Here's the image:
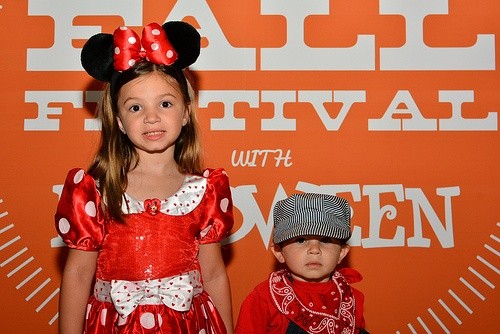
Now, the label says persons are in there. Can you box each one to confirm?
[54,21,234,334]
[234,193,372,334]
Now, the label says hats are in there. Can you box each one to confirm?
[273,193,351,244]
